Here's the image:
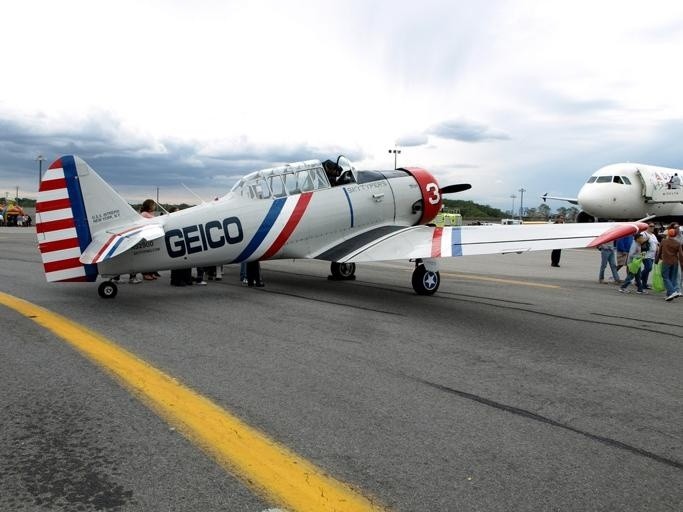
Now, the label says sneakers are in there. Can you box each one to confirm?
[112,271,160,283]
[599,279,653,295]
[665,291,682,301]
[197,272,224,285]
[243,278,264,288]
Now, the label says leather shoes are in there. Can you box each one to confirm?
[552,263,560,267]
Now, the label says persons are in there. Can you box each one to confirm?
[596,216,683,301]
[103,196,268,288]
[6,214,32,228]
[550,214,565,268]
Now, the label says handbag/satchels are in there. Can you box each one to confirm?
[642,240,650,252]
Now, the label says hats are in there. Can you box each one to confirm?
[668,229,675,236]
[633,234,643,240]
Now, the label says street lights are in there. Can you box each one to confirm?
[509,193,516,215]
[32,155,47,188]
[518,188,526,219]
[388,149,401,170]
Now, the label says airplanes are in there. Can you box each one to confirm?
[536,163,683,228]
[30,153,653,299]
